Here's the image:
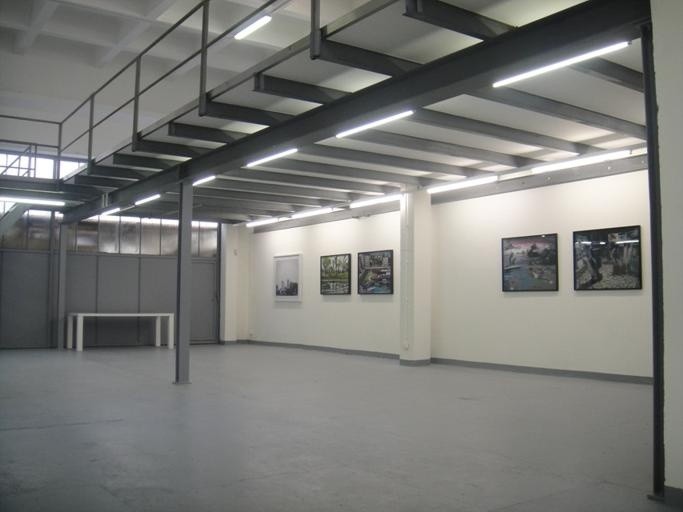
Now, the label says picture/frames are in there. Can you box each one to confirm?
[319,253,350,296]
[572,224,642,292]
[500,232,559,294]
[356,250,394,296]
[273,253,302,302]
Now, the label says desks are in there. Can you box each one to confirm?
[66,310,175,353]
